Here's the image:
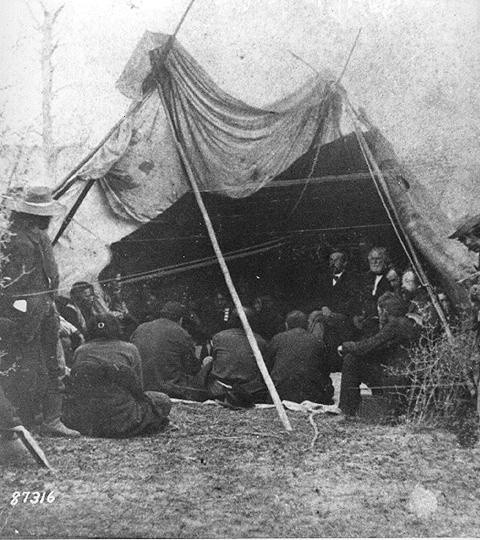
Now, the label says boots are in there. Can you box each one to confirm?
[39,391,81,439]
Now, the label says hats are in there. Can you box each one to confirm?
[6,182,67,217]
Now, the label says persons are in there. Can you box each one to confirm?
[56,264,269,439]
[267,246,452,423]
[2,186,81,439]
